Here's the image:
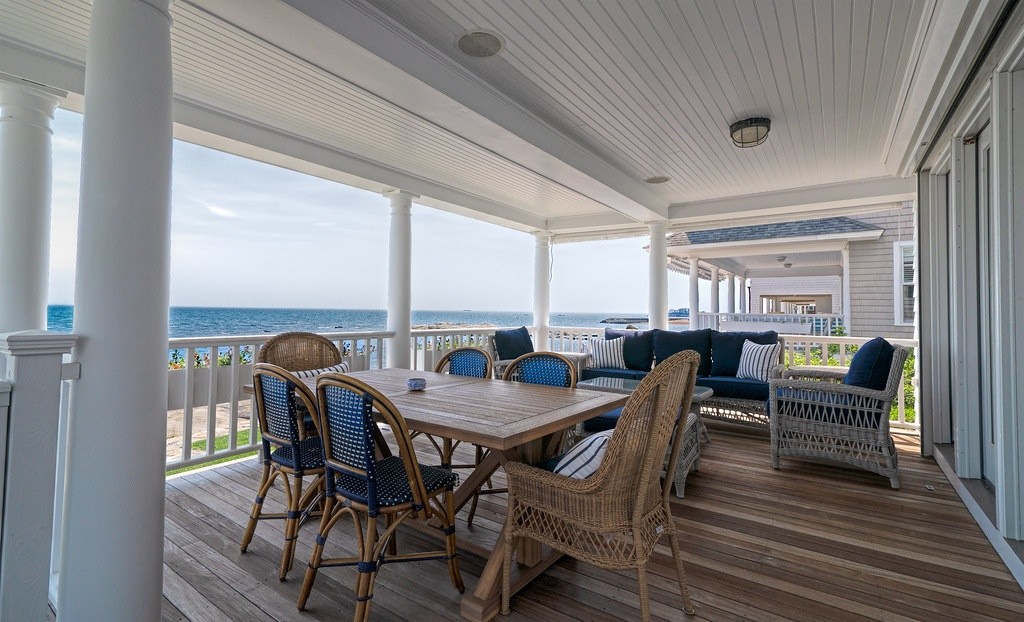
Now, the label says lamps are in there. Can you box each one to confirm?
[730,117,771,148]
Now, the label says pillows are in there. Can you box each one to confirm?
[556,427,616,480]
[736,338,782,383]
[589,335,628,371]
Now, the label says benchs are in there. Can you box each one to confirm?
[574,324,786,440]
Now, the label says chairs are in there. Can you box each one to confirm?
[498,349,702,622]
[486,327,586,451]
[769,336,908,491]
[240,331,398,583]
[399,345,492,506]
[466,351,577,527]
[298,372,466,622]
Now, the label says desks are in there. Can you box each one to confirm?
[242,368,632,622]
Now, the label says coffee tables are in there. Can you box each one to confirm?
[575,376,714,458]
